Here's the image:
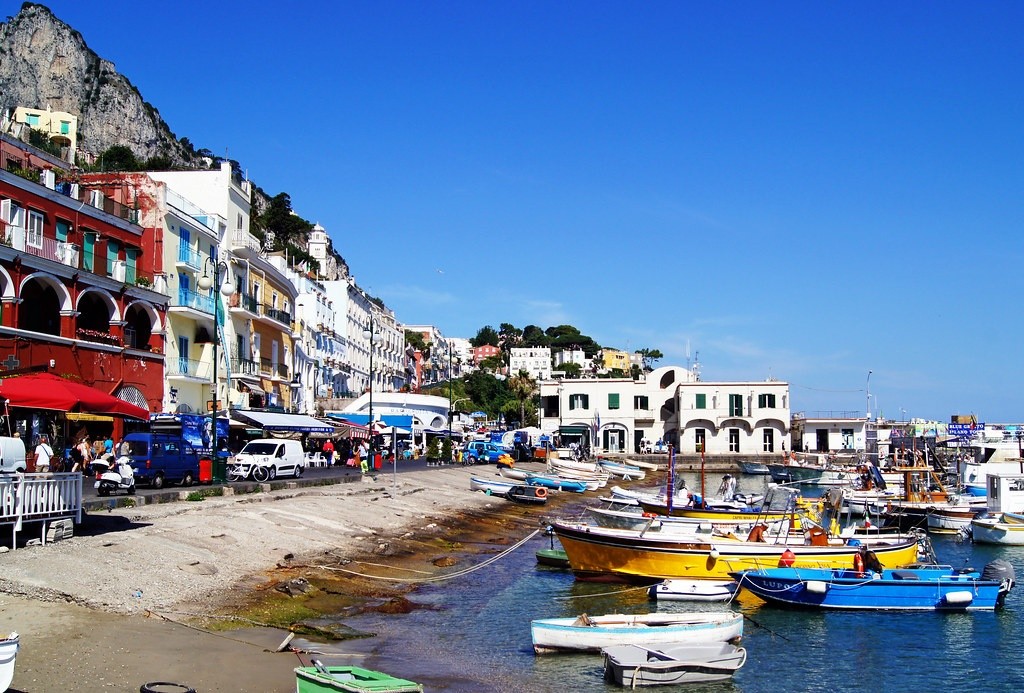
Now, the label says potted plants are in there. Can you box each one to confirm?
[135,275,150,289]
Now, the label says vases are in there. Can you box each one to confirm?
[76,336,118,345]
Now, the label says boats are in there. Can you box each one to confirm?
[534,434,1024,578]
[597,642,747,684]
[529,610,743,653]
[469,477,548,503]
[727,561,1001,614]
[290,647,425,692]
[647,578,740,601]
[498,454,660,493]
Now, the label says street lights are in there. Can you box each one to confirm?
[443,341,458,441]
[196,256,235,485]
[361,314,382,472]
[865,369,873,420]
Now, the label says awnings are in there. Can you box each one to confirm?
[238,411,334,433]
[242,380,265,396]
[561,429,582,436]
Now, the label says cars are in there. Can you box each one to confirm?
[462,441,509,465]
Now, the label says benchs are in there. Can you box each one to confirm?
[892,571,919,580]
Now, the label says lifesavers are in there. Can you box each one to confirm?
[642,513,657,517]
[853,553,864,578]
[535,488,547,497]
[515,487,525,495]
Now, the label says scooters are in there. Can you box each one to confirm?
[90,449,137,496]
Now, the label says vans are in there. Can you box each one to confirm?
[115,432,200,487]
[232,439,306,481]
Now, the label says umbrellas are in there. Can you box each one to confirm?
[0,373,149,421]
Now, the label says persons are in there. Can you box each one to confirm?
[640,436,663,455]
[554,438,558,447]
[782,440,785,450]
[71,435,122,472]
[686,493,711,510]
[805,442,809,453]
[33,437,54,479]
[353,439,369,468]
[477,446,490,464]
[574,444,590,462]
[409,444,420,460]
[323,440,334,468]
[451,442,469,466]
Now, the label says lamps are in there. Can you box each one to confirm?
[169,389,178,403]
[69,225,73,231]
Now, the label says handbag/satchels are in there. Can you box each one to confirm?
[83,467,93,476]
[70,445,83,463]
[116,442,122,457]
[111,445,116,458]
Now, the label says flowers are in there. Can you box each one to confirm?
[76,328,119,341]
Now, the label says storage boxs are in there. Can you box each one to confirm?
[92,459,110,471]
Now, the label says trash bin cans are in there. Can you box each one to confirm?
[372,451,382,470]
[216,456,228,479]
[200,456,213,485]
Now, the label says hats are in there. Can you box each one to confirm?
[41,438,46,443]
[687,493,692,498]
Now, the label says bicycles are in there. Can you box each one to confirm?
[224,451,270,483]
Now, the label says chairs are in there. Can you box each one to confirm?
[305,452,327,469]
